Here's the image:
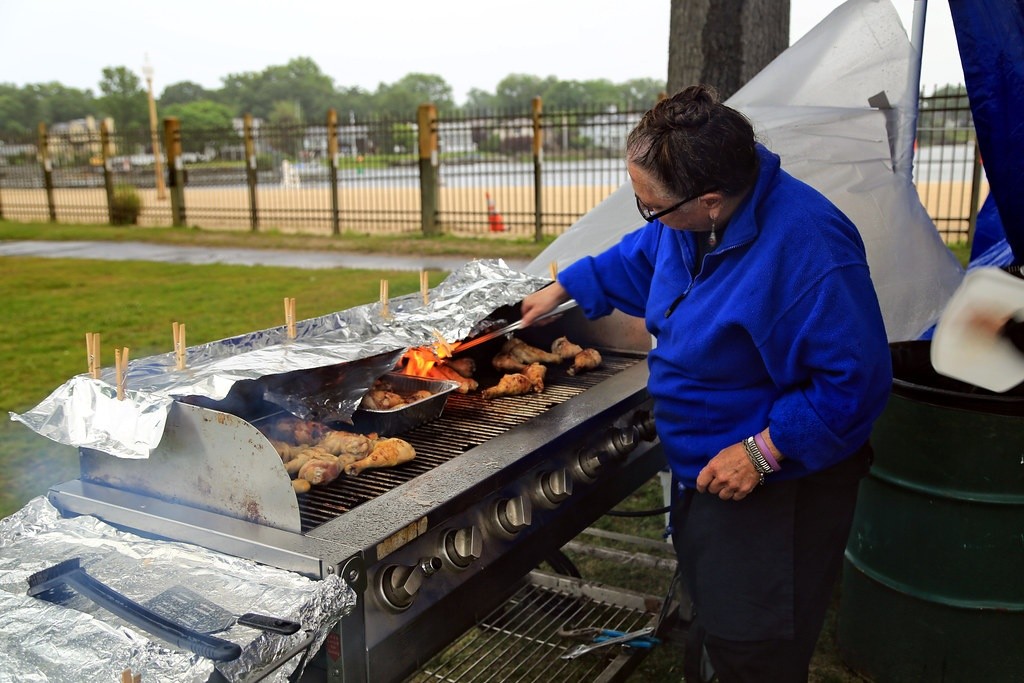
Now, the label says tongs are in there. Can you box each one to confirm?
[449,294,576,356]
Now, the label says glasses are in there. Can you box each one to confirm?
[635,186,726,224]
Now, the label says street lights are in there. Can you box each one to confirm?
[142,52,167,201]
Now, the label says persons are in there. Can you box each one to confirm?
[521,87,891,683]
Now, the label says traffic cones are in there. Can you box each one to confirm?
[485,191,510,235]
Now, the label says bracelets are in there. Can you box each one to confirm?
[742,433,781,485]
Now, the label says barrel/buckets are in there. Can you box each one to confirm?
[831,338,1024,683]
